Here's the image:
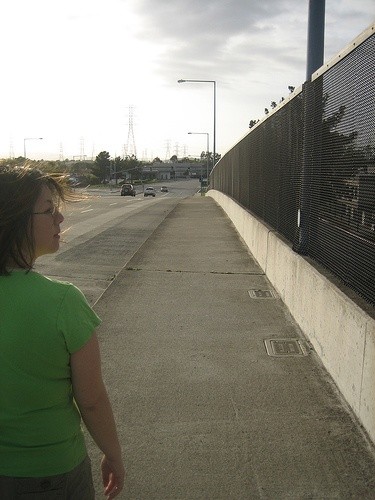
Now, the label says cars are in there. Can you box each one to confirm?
[144,187,156,197]
[160,186,168,192]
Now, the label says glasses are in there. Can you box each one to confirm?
[31,205,58,216]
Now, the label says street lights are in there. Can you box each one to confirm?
[178,79,216,165]
[187,133,209,185]
[23,138,44,158]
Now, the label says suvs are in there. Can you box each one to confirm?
[120,184,135,196]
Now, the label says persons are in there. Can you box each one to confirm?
[0,163,123,500]
